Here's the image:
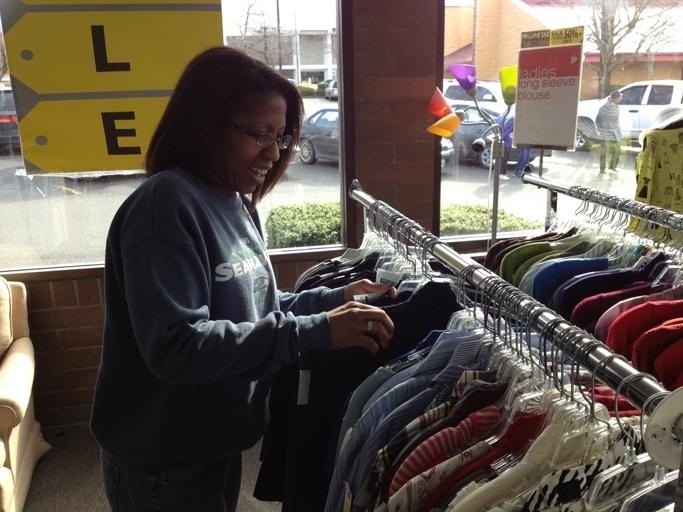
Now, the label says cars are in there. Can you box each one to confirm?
[297,73,530,173]
[0,81,23,158]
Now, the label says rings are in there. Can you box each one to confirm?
[366,319,376,334]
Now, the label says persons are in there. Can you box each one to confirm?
[89,46,399,510]
[593,90,625,178]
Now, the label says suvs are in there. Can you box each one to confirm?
[569,76,682,153]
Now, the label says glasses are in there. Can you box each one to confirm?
[228,120,293,151]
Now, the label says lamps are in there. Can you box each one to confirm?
[426,63,520,245]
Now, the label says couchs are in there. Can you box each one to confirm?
[1,274,52,512]
